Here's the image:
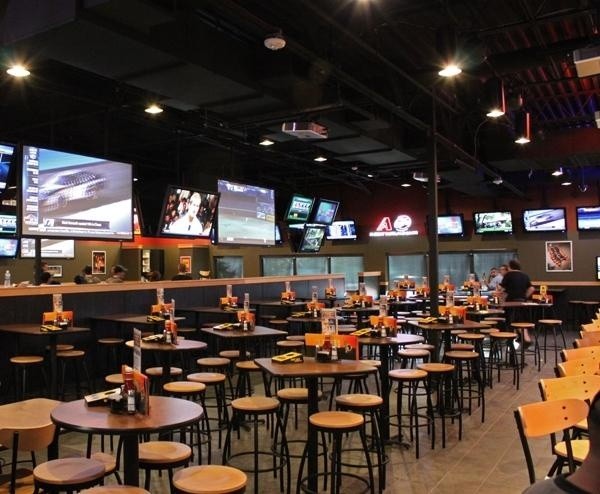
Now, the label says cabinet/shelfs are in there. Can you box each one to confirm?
[0,274,346,297]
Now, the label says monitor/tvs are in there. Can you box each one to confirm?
[214,174,277,249]
[19,238,36,261]
[520,208,567,234]
[0,235,18,259]
[438,213,465,237]
[310,197,340,226]
[1,214,16,234]
[324,220,359,242]
[471,210,514,236]
[16,140,135,243]
[156,184,221,240]
[295,225,325,252]
[40,238,75,260]
[282,193,315,224]
[576,205,600,231]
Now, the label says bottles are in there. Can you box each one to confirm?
[240,313,245,327]
[163,329,166,339]
[449,314,454,324]
[124,367,137,394]
[119,384,127,410]
[315,345,320,359]
[377,316,384,337]
[445,308,450,320]
[126,388,136,414]
[164,320,171,333]
[322,335,332,355]
[4,270,11,287]
[243,320,248,331]
[313,308,317,317]
[165,330,172,343]
[331,345,338,360]
[380,325,387,337]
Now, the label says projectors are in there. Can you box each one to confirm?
[413,172,442,186]
[279,121,329,141]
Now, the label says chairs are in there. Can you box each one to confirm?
[578,330,600,339]
[572,337,600,349]
[598,308,600,312]
[0,398,63,494]
[553,357,598,439]
[538,374,600,480]
[595,312,600,318]
[581,324,600,332]
[513,398,591,485]
[592,318,600,324]
[560,345,599,367]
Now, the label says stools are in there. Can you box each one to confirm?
[300,411,375,493]
[359,359,382,397]
[259,314,276,325]
[510,322,540,373]
[219,349,252,422]
[484,317,506,330]
[480,320,498,325]
[388,368,436,459]
[396,319,407,324]
[56,350,91,398]
[46,344,74,351]
[222,396,291,494]
[489,332,519,390]
[277,387,323,430]
[397,315,405,319]
[333,393,391,494]
[276,341,304,355]
[233,359,283,430]
[158,381,212,465]
[172,464,248,494]
[177,326,196,340]
[457,333,486,388]
[202,322,215,328]
[338,327,357,335]
[185,372,229,449]
[439,330,467,343]
[197,357,240,440]
[451,343,480,407]
[410,362,463,449]
[337,324,356,328]
[397,325,402,334]
[396,349,430,368]
[97,337,124,371]
[6,355,50,400]
[535,319,567,366]
[141,331,155,337]
[90,451,123,485]
[268,320,289,330]
[137,441,196,491]
[403,344,435,364]
[285,335,307,356]
[32,457,105,494]
[440,351,486,423]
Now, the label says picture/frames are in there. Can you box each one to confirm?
[179,256,191,273]
[545,241,573,273]
[92,251,106,274]
[596,256,600,280]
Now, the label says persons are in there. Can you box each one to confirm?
[500,258,535,343]
[170,193,203,233]
[40,260,48,271]
[496,264,508,291]
[104,264,127,283]
[520,389,600,494]
[171,263,192,280]
[483,267,503,291]
[38,272,51,286]
